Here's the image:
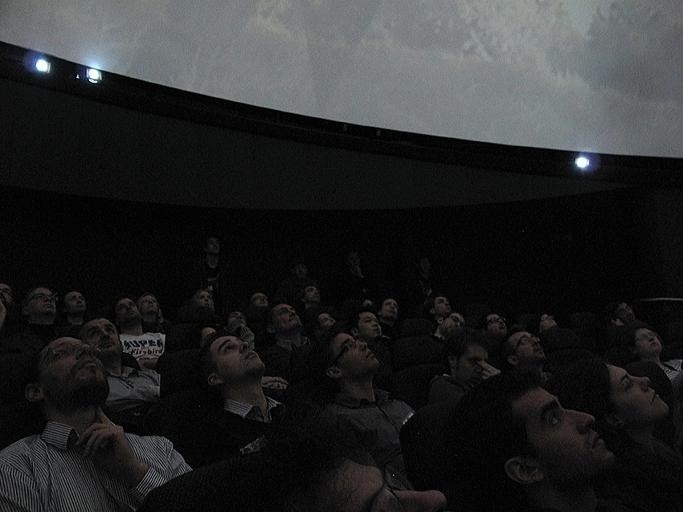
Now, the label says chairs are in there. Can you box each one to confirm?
[0,299,682,511]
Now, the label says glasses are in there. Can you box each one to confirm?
[333,338,365,364]
[367,466,409,512]
[487,317,506,324]
[513,335,540,352]
[24,293,55,307]
